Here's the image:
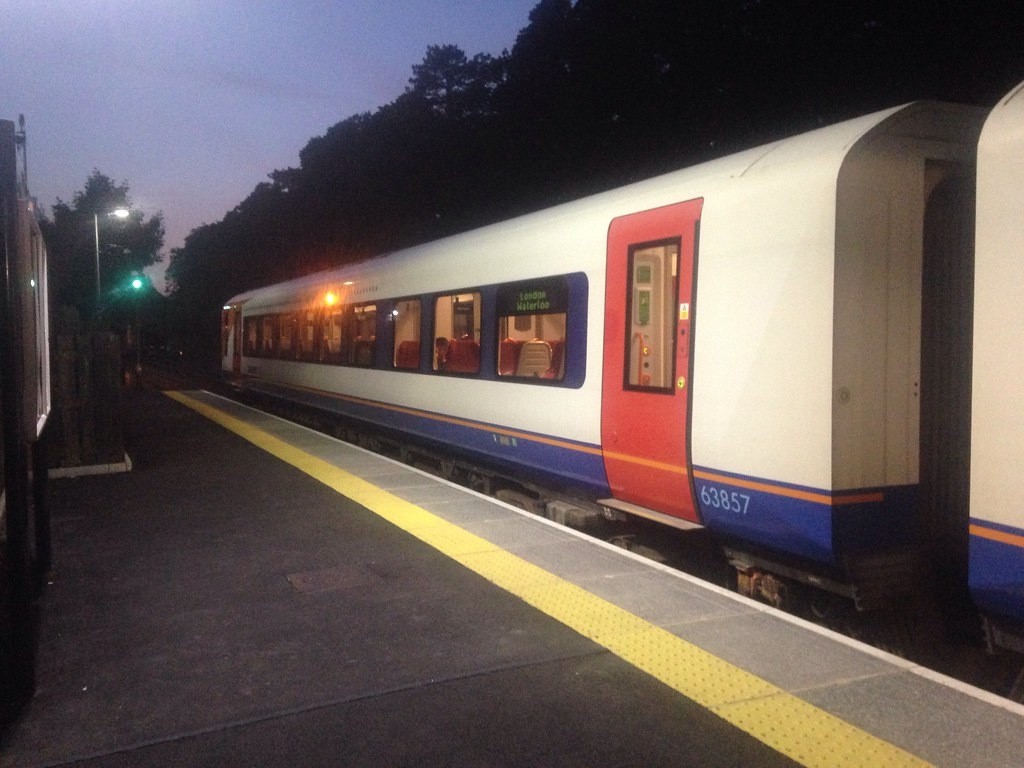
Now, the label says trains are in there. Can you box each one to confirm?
[222,78,1024,705]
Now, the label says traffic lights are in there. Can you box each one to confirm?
[133,277,145,314]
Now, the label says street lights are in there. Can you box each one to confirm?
[91,208,130,344]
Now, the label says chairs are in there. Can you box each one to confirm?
[501,339,524,375]
[513,341,553,378]
[444,341,478,373]
[247,340,373,365]
[397,341,420,369]
